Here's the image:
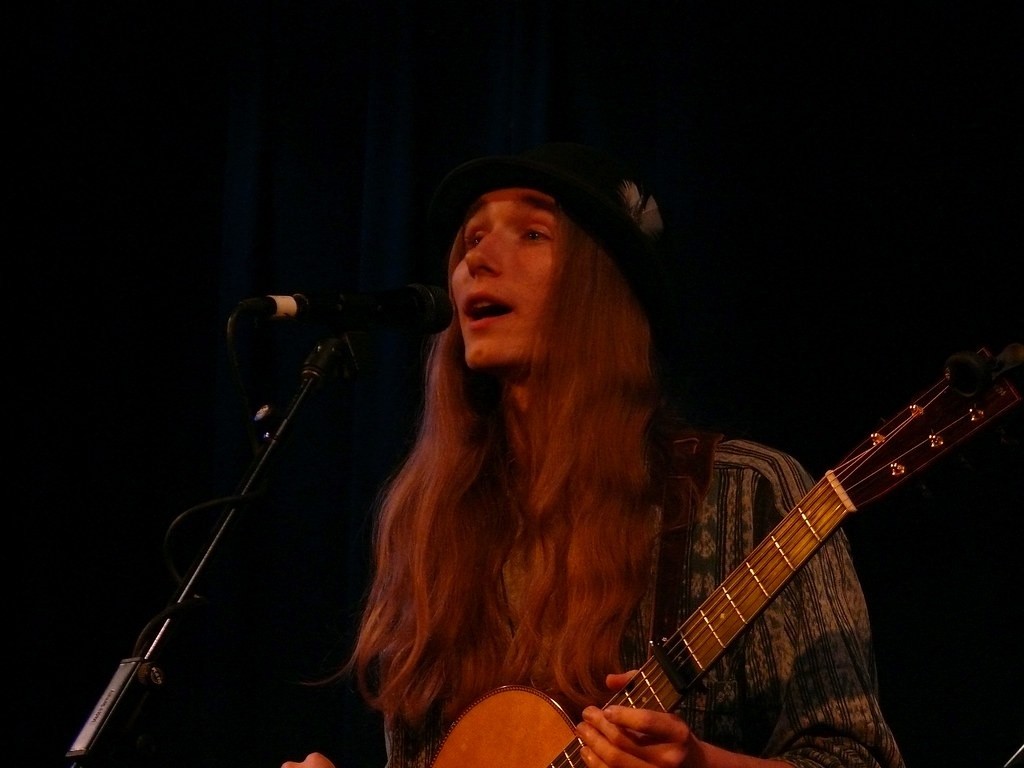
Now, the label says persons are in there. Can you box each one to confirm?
[276,142,906,768]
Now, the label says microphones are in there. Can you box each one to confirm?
[244,283,454,337]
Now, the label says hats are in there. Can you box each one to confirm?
[429,145,672,293]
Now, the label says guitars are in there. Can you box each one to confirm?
[427,332,1022,768]
